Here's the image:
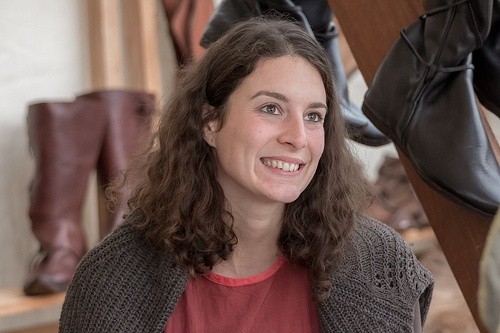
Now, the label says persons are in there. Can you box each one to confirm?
[58,16,437,333]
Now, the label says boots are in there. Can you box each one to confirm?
[199,0,393,147]
[23,101,108,295]
[361,0,500,218]
[76,90,156,242]
